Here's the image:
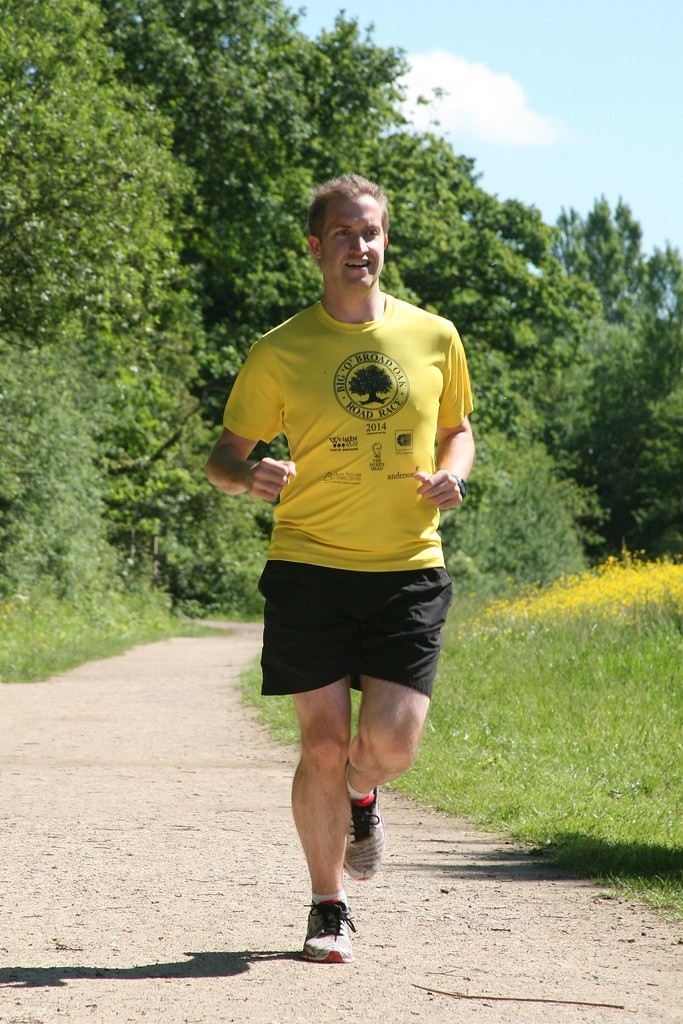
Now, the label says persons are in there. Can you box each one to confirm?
[203,171,479,964]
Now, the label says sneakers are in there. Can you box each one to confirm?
[302,901,355,964]
[342,756,385,881]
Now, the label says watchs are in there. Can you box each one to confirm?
[447,472,468,501]
